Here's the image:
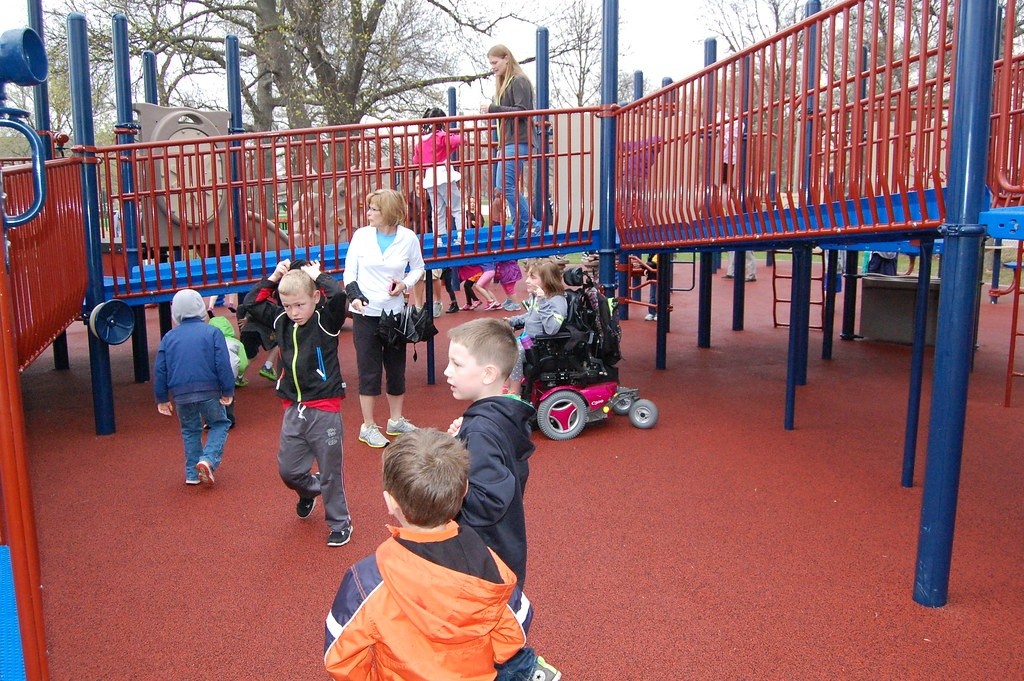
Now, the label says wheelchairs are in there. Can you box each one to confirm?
[509,267,660,442]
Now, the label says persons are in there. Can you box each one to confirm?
[699,105,745,216]
[444,318,538,592]
[153,289,235,485]
[824,250,916,292]
[644,254,657,321]
[322,427,562,681]
[244,258,353,546]
[504,258,568,398]
[721,250,757,282]
[482,44,542,239]
[202,292,280,433]
[412,107,468,247]
[405,172,619,318]
[618,135,662,227]
[343,188,426,448]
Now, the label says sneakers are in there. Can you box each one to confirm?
[386,416,419,435]
[327,526,353,545]
[296,471,320,518]
[359,423,390,447]
[234,373,248,387]
[259,365,277,380]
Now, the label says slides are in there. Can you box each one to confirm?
[240,205,353,319]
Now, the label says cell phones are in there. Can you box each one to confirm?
[387,281,396,291]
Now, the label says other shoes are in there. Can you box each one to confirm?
[207,306,215,318]
[186,479,200,484]
[470,298,482,310]
[745,277,757,282]
[501,298,521,310]
[644,314,657,320]
[484,300,501,310]
[505,225,541,237]
[196,458,214,484]
[526,655,562,681]
[228,302,237,312]
[459,303,470,310]
[446,302,459,313]
[433,301,443,317]
[722,274,734,280]
